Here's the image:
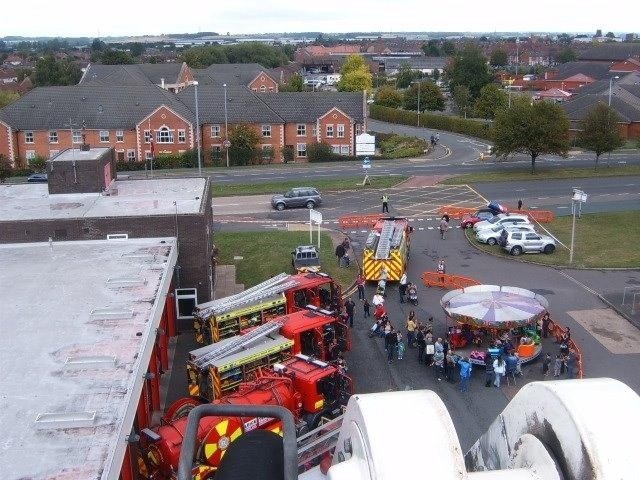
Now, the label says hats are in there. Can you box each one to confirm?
[302,71,376,106]
[461,207,499,229]
[28,172,47,182]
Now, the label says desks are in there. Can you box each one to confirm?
[507,77,516,109]
[569,189,588,261]
[223,83,231,167]
[608,76,621,121]
[193,81,203,171]
[411,81,420,127]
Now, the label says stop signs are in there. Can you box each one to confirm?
[362,214,412,285]
[186,303,354,404]
[192,265,351,346]
[136,350,352,479]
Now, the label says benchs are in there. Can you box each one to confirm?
[150,129,154,152]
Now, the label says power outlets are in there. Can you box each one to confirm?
[291,245,321,272]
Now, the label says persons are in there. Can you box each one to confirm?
[334,239,578,393]
[439,217,448,240]
[441,212,449,232]
[380,193,389,213]
[430,133,440,141]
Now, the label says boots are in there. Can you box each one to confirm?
[499,227,557,255]
[476,221,536,246]
[472,212,530,231]
[271,187,323,211]
